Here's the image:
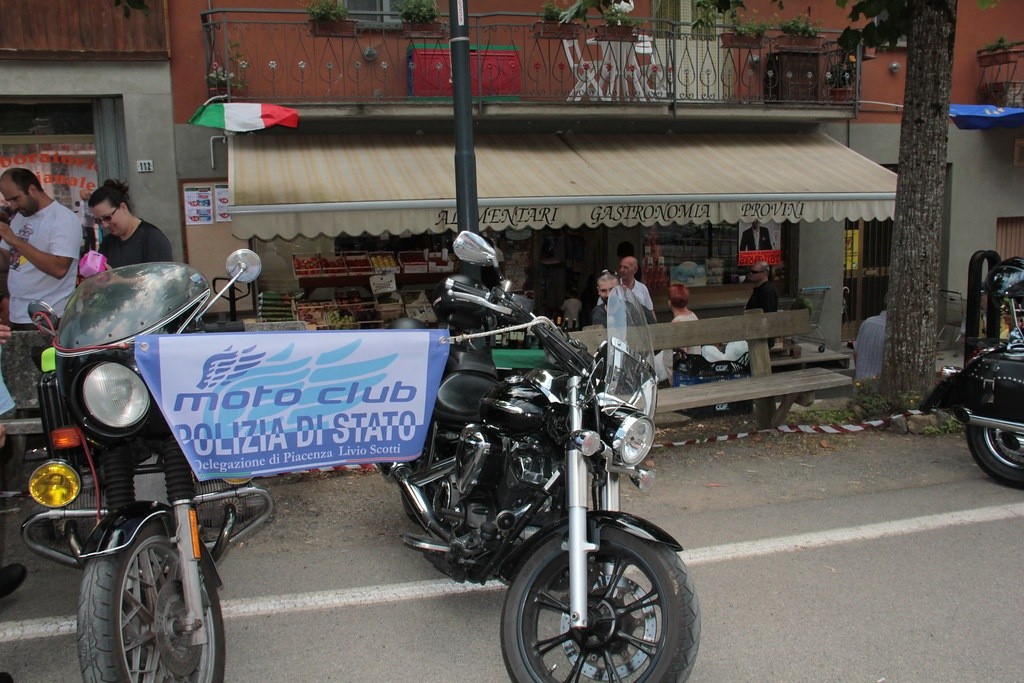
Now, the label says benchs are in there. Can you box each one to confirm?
[565,304,854,437]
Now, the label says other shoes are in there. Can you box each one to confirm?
[0,564,26,600]
[0,672,13,683]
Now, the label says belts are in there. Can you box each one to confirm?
[8,322,37,329]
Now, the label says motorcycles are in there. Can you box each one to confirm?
[918,249,1024,489]
[20,248,273,683]
[376,230,702,683]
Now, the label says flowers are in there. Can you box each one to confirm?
[202,59,231,84]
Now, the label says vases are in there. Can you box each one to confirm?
[206,85,232,104]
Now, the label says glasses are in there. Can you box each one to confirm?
[751,270,764,274]
[95,207,118,224]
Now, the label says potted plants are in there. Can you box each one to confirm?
[774,15,825,51]
[390,0,442,34]
[304,0,358,36]
[532,2,581,39]
[591,2,647,42]
[718,9,765,48]
[828,62,857,102]
[974,33,1023,67]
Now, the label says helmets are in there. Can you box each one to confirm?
[985,256,1024,315]
[432,274,489,324]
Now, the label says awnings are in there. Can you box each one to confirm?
[228,126,897,243]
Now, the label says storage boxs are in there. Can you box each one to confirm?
[289,251,455,329]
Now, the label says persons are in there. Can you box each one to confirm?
[740,220,772,251]
[0,324,28,683]
[523,267,538,293]
[89,179,181,336]
[561,290,582,330]
[571,284,592,321]
[0,168,83,330]
[619,256,657,324]
[81,192,102,259]
[662,284,701,387]
[745,261,778,349]
[590,273,642,329]
[853,291,888,394]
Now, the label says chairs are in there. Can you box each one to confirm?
[560,34,653,103]
